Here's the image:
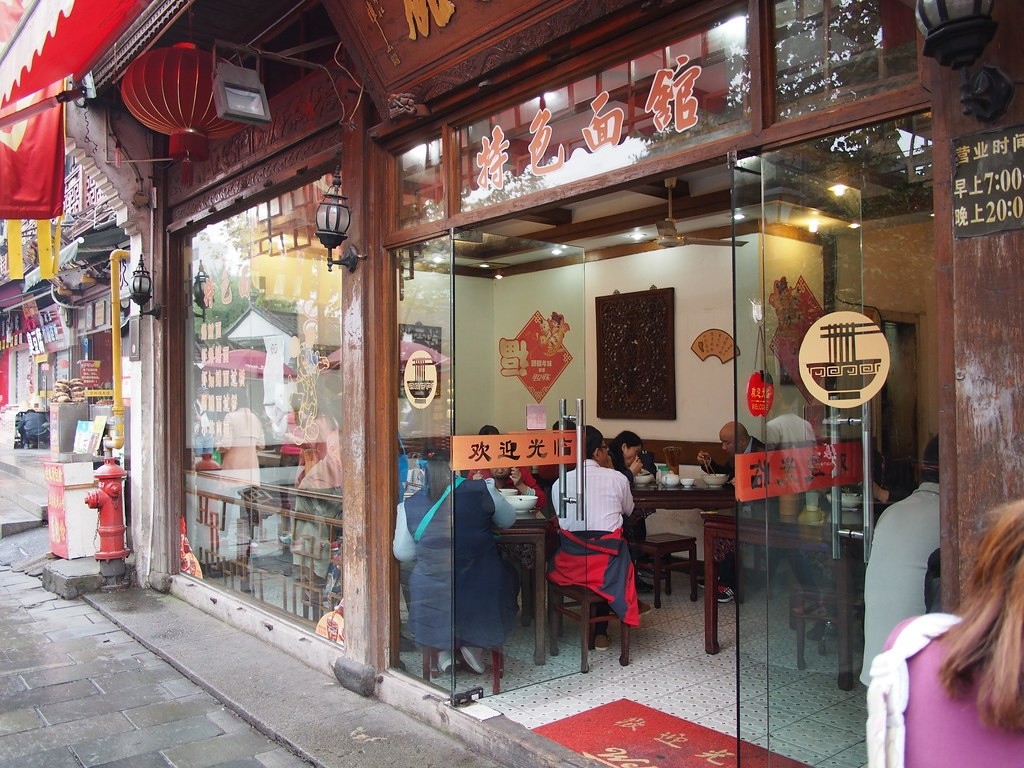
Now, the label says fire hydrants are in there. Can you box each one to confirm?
[84,456,132,592]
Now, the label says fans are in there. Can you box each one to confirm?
[605,176,748,252]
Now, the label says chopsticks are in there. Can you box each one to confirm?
[700,449,717,476]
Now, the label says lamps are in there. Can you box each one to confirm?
[126,253,161,321]
[211,39,353,127]
[915,0,1016,124]
[313,165,357,275]
[0,234,36,257]
[63,213,99,225]
[7,310,12,344]
[190,258,215,323]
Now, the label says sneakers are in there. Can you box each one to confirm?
[636,578,653,593]
[698,578,705,588]
[718,587,735,602]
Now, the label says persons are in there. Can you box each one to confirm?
[696,422,765,604]
[872,446,907,503]
[16,408,50,449]
[761,376,820,611]
[269,393,305,514]
[290,428,344,582]
[468,466,546,613]
[393,450,516,674]
[882,498,1024,768]
[285,388,342,546]
[607,430,654,594]
[552,425,652,650]
[193,387,214,457]
[214,387,266,544]
[477,424,500,436]
[858,433,942,689]
[537,420,576,479]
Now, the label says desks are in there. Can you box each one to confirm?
[236,478,295,593]
[700,500,886,691]
[622,485,735,547]
[491,510,547,666]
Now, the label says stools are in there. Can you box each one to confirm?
[793,577,868,672]
[422,636,504,695]
[632,533,697,609]
[547,582,630,674]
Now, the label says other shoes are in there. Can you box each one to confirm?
[436,649,460,674]
[23,443,29,448]
[461,645,485,674]
[594,635,609,651]
[806,619,838,656]
[640,600,651,617]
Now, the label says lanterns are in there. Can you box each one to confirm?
[120,41,248,159]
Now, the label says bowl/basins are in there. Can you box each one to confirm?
[701,474,729,487]
[497,489,538,513]
[825,492,863,508]
[680,479,695,488]
[633,475,654,486]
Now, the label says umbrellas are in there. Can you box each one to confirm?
[202,346,299,380]
[317,339,453,375]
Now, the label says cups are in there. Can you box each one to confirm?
[805,491,818,512]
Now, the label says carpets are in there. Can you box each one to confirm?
[528,697,818,768]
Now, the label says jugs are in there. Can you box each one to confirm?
[661,470,679,488]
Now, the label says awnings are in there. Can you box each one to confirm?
[0,0,183,220]
[22,228,131,295]
